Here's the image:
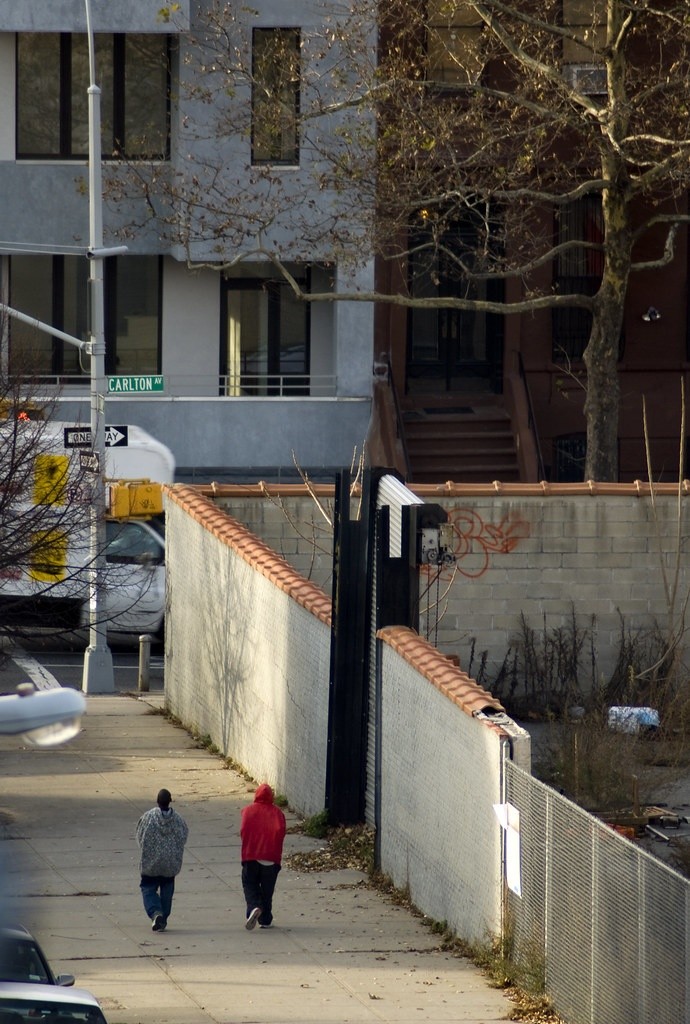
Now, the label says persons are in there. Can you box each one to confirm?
[136,789,188,932]
[240,784,287,930]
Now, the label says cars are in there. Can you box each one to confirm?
[1,922,76,987]
[1,982,107,1024]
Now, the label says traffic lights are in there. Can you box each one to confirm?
[108,486,130,518]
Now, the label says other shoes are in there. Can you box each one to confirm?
[246,907,262,930]
[260,922,272,928]
[157,927,165,931]
[152,914,164,931]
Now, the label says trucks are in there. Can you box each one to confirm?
[0,418,175,647]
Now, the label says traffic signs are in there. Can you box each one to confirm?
[63,426,129,448]
